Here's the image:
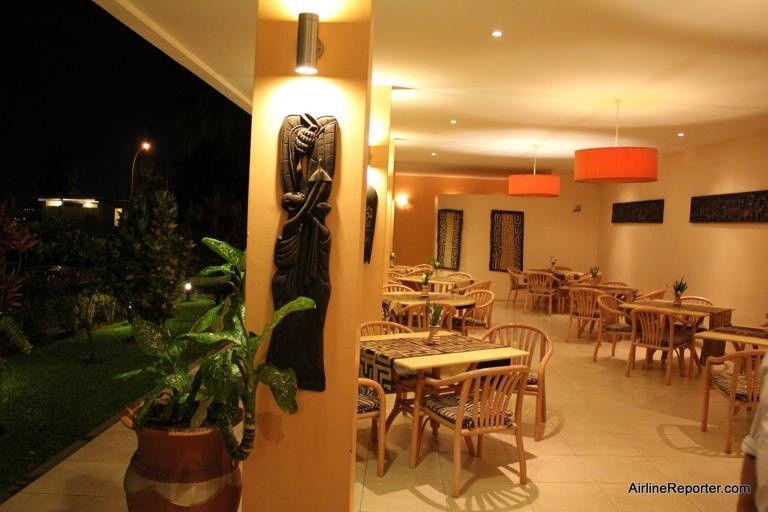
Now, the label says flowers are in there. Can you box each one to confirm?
[549,255,559,263]
[431,259,444,267]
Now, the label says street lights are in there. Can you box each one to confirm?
[129,140,154,197]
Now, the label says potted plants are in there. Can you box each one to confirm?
[109,236,318,512]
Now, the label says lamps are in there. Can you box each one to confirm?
[574,99,658,184]
[294,13,324,73]
[508,145,561,198]
